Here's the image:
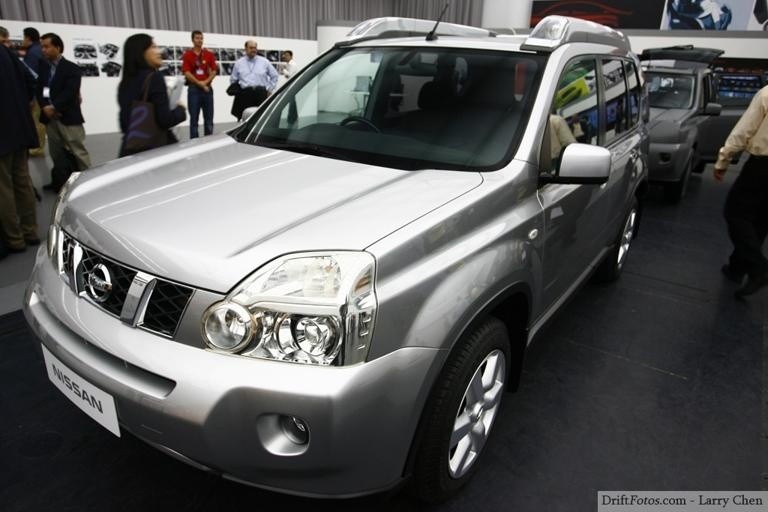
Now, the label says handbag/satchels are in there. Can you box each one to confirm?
[124,72,179,152]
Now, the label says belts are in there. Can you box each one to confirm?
[242,86,266,91]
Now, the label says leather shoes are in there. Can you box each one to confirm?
[720,264,744,284]
[733,258,768,299]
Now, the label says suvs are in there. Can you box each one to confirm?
[636,42,751,198]
[25,16,648,501]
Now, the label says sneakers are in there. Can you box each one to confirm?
[6,245,27,254]
[25,239,41,245]
[43,184,53,190]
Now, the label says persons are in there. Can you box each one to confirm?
[227,38,281,123]
[116,33,187,158]
[182,31,217,141]
[277,50,296,79]
[713,84,768,296]
[545,116,579,162]
[1,27,91,261]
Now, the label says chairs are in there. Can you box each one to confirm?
[386,80,451,146]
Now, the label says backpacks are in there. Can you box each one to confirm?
[51,164,73,194]
